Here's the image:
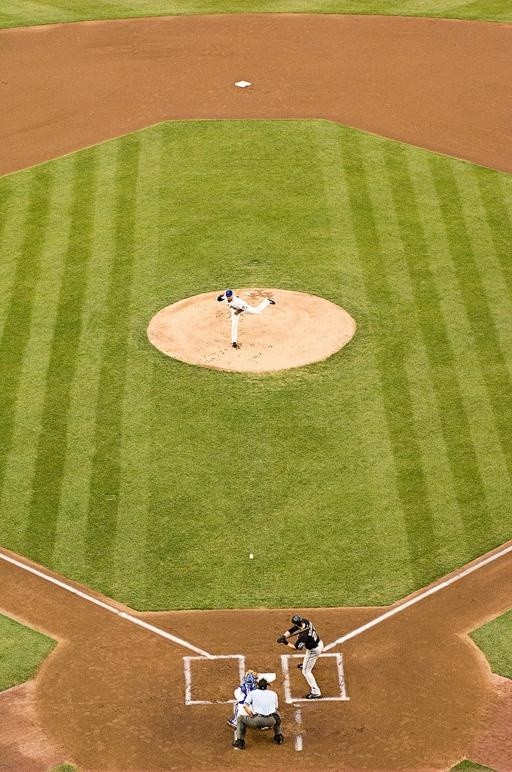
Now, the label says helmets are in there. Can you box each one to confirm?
[258,679,267,689]
[292,615,302,623]
[225,291,232,297]
[244,674,256,689]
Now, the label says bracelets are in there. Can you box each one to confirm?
[284,639,289,645]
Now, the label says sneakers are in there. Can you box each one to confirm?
[226,719,237,729]
[233,741,244,748]
[303,693,321,698]
[269,299,275,304]
[275,736,283,744]
[298,664,303,668]
[233,342,237,347]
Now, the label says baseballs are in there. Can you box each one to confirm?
[249,554,253,559]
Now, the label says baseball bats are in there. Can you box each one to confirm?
[284,627,310,639]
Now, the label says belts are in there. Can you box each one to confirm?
[257,713,271,717]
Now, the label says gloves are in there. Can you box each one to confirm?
[277,636,288,645]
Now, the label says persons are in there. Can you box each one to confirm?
[280,615,324,699]
[217,290,275,348]
[227,673,270,730]
[232,679,284,750]
